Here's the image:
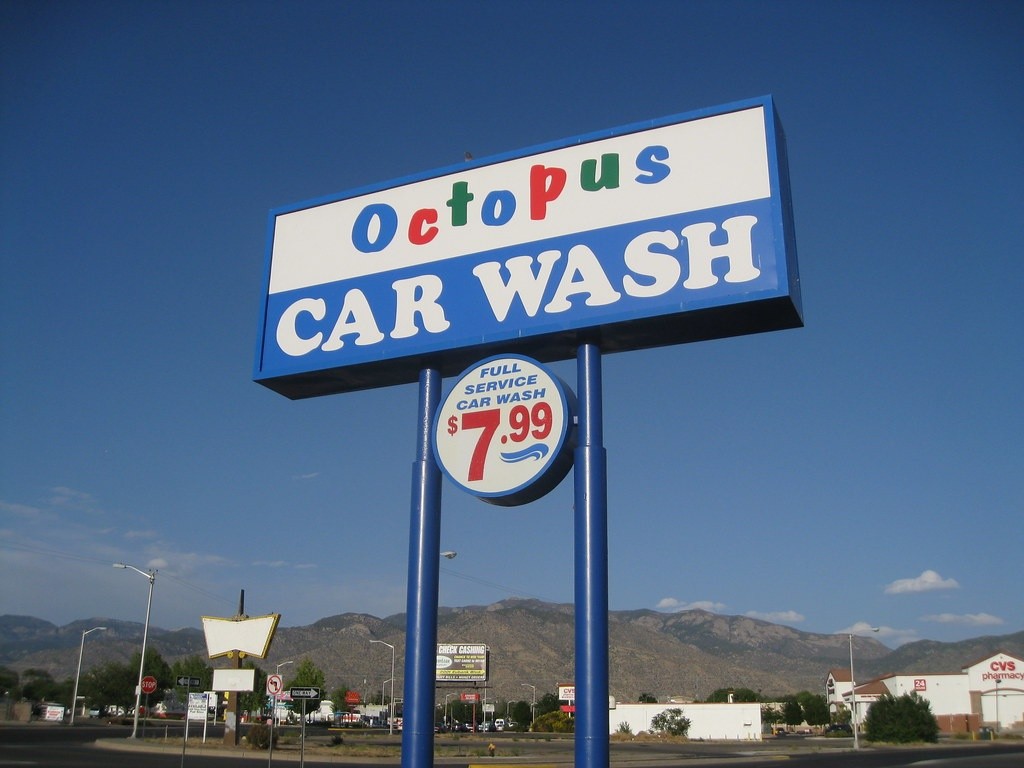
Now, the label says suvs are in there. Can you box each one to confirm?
[824,724,852,737]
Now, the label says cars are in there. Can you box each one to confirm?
[434,718,518,734]
[775,727,785,734]
[89,706,103,719]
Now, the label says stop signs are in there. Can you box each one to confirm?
[140,676,157,694]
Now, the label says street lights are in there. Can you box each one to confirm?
[275,661,294,728]
[69,626,107,727]
[112,563,158,739]
[849,627,880,750]
[994,678,1001,733]
[382,678,397,728]
[506,700,515,715]
[444,692,457,726]
[369,640,394,736]
[521,683,535,726]
[481,698,492,722]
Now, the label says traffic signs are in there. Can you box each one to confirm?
[177,675,201,687]
[290,687,320,699]
